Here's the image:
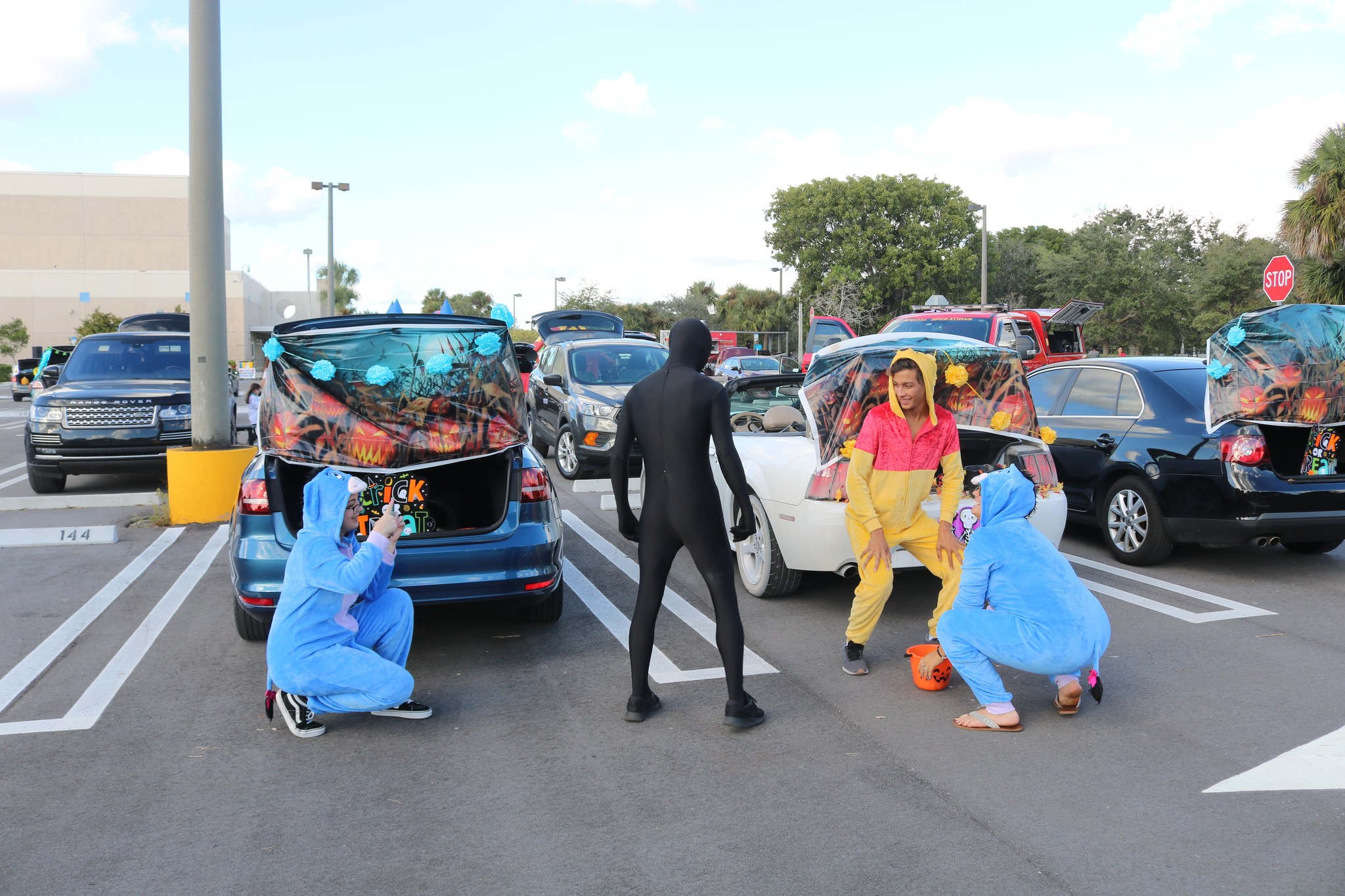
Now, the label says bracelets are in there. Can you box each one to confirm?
[937,646,947,659]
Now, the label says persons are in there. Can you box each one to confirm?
[1087,344,1101,358]
[746,340,762,356]
[703,364,722,376]
[266,467,433,737]
[610,318,765,726]
[841,348,966,675]
[245,358,270,446]
[1117,347,1127,357]
[918,462,1111,731]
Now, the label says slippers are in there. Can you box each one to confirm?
[953,708,1024,732]
[1054,693,1081,715]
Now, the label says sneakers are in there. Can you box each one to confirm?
[370,699,432,719]
[626,691,662,721]
[840,639,870,675]
[276,689,326,739]
[723,690,765,727]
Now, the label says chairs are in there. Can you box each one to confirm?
[758,405,803,433]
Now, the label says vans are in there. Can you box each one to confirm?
[803,295,1105,376]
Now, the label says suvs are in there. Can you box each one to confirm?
[24,311,238,495]
[525,309,669,480]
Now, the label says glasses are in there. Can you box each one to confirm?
[345,496,365,513]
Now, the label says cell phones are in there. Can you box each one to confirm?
[390,497,394,517]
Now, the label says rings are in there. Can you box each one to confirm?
[924,667,928,670]
[920,659,922,663]
[922,663,925,667]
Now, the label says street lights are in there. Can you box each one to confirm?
[513,293,522,330]
[554,277,566,311]
[312,181,349,317]
[303,248,312,318]
[771,268,782,298]
[525,321,532,331]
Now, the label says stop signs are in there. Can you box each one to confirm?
[1263,255,1294,302]
[809,306,815,326]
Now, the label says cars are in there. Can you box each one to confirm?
[702,346,807,404]
[512,342,544,400]
[997,304,1345,568]
[29,346,76,407]
[11,358,42,402]
[709,329,1068,601]
[227,313,564,642]
[227,365,239,397]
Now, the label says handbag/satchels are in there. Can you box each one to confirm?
[1300,421,1342,476]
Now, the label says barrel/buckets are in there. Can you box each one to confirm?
[904,644,952,690]
[964,464,1004,498]
